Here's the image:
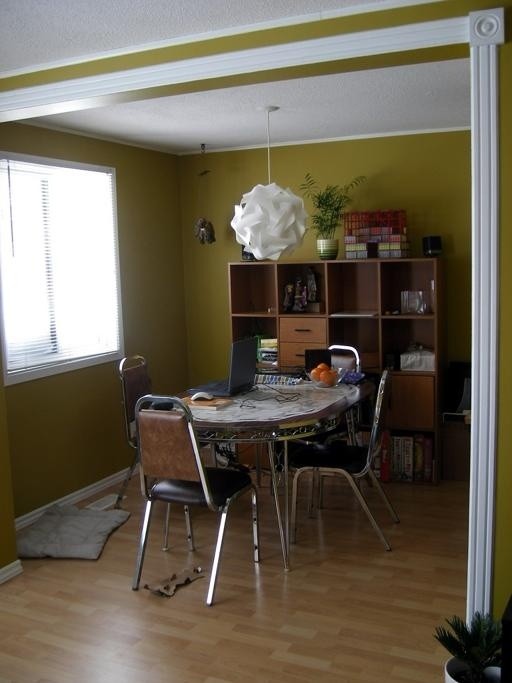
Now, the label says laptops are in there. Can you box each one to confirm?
[187,336,259,397]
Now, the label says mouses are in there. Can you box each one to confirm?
[190,391,214,401]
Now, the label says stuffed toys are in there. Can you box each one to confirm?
[193,216,217,243]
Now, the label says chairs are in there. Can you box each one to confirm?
[129,394,261,607]
[269,344,360,495]
[290,367,398,551]
[115,354,149,510]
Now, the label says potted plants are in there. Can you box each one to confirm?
[432,612,503,683]
[298,168,370,260]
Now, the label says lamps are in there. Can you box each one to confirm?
[231,105,308,263]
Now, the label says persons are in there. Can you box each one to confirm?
[305,265,318,302]
[292,276,304,311]
[282,280,295,310]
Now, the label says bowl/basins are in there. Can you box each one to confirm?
[341,370,366,385]
[305,367,347,387]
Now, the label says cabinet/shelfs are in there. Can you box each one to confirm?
[227,258,443,486]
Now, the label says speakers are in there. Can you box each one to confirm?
[305,349,331,371]
[422,235,443,256]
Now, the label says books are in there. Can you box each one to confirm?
[174,395,233,410]
[259,336,278,365]
[347,428,434,483]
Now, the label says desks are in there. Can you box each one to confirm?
[165,376,362,572]
